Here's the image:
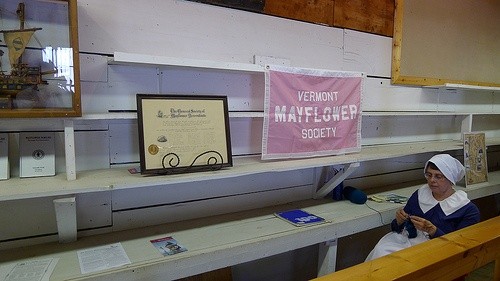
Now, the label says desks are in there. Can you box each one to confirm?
[0,171,500,281]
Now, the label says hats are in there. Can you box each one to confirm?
[424,154,465,185]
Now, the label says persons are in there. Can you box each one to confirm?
[363,152,482,281]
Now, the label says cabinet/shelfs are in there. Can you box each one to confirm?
[0,52,500,241]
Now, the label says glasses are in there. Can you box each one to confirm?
[425,173,446,182]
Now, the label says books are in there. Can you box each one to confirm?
[273,209,326,227]
